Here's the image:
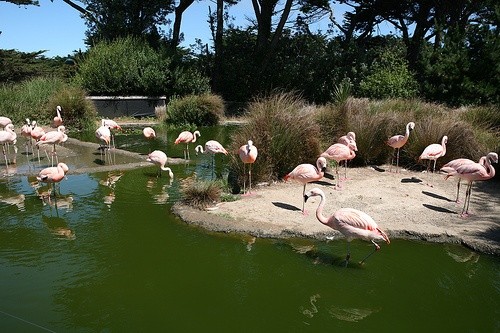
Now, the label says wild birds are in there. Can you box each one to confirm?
[418,134,448,188]
[93,126,111,157]
[319,141,357,190]
[101,118,123,151]
[20,117,34,158]
[0,123,18,159]
[440,155,491,205]
[443,151,499,219]
[35,162,70,196]
[194,140,228,180]
[337,131,356,181]
[234,139,258,197]
[30,121,50,162]
[142,150,174,179]
[303,187,390,270]
[39,132,68,162]
[142,127,157,140]
[0,115,13,131]
[173,129,201,160]
[283,156,328,216]
[384,121,416,174]
[53,105,62,127]
[34,125,66,167]
